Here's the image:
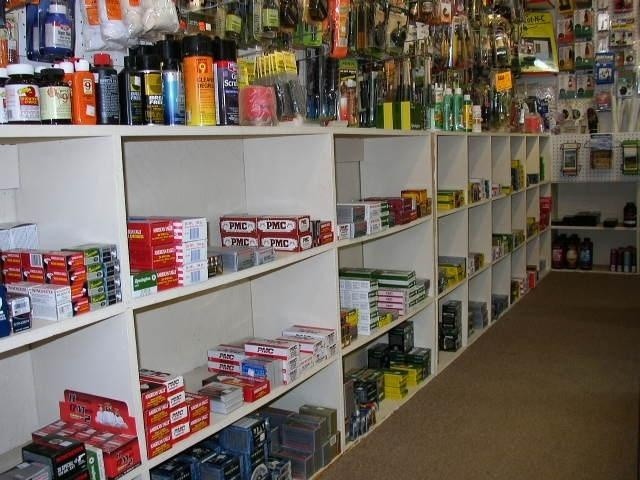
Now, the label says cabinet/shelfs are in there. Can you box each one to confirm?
[432,131,491,377]
[2,125,343,479]
[552,223,640,275]
[491,133,552,325]
[334,128,435,429]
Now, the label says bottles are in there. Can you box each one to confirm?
[0,1,238,127]
[552,233,638,274]
[385,57,490,133]
[624,200,637,227]
